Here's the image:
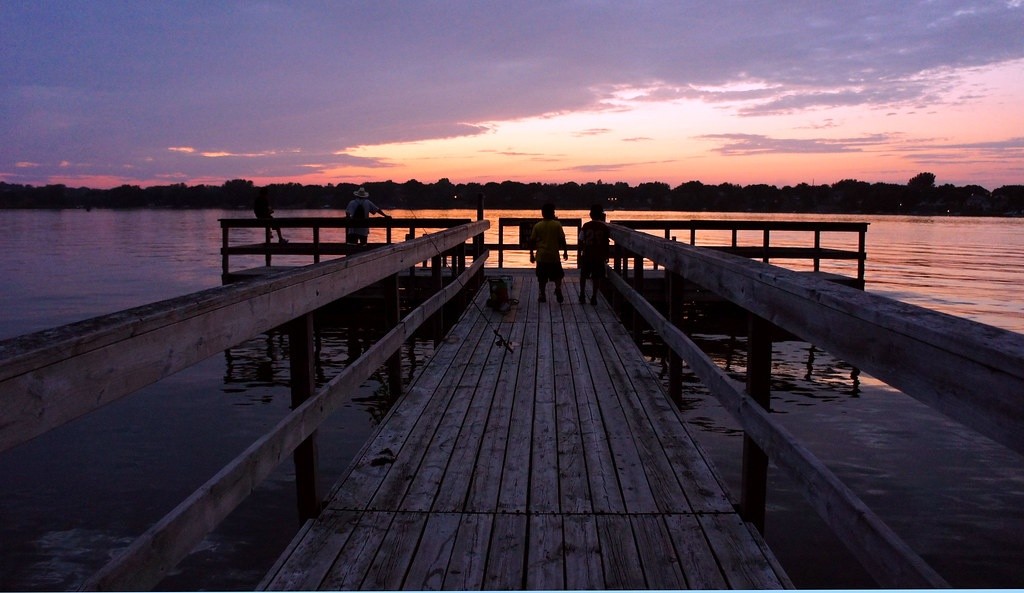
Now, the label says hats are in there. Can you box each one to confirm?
[354,189,369,198]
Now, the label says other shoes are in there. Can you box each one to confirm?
[538,296,546,302]
[555,288,564,302]
[579,295,587,304]
[590,298,597,305]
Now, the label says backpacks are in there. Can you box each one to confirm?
[353,199,366,218]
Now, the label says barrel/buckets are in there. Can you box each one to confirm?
[488,275,513,305]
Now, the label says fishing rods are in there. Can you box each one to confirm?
[406,203,514,355]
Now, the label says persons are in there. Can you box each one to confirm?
[346,187,392,256]
[528,203,568,303]
[253,188,289,244]
[577,206,609,305]
